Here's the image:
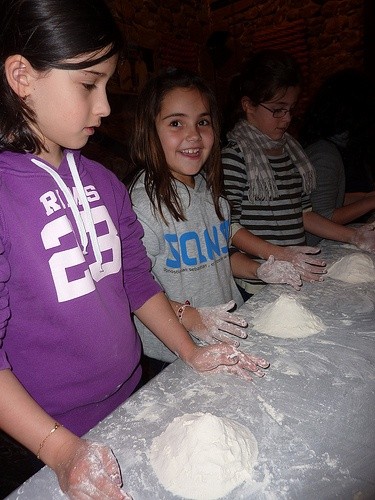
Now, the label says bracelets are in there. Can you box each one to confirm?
[36,422,64,461]
[176,300,190,323]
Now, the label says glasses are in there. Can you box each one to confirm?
[250,98,298,118]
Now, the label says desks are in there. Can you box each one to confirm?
[4,223,375,500]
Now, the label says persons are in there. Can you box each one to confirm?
[125,72,302,382]
[0,0,270,500]
[81,129,130,180]
[296,69,375,246]
[221,49,375,283]
